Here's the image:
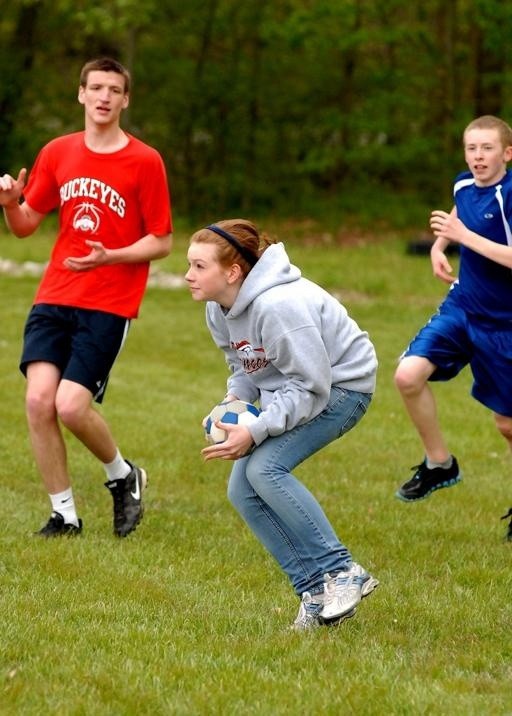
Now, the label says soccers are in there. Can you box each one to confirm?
[205,400,260,458]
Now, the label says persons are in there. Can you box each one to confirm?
[390,111,510,547]
[2,56,172,544]
[180,215,381,632]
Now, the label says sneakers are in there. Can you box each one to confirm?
[284,563,380,632]
[28,511,84,539]
[394,454,464,504]
[101,458,150,539]
